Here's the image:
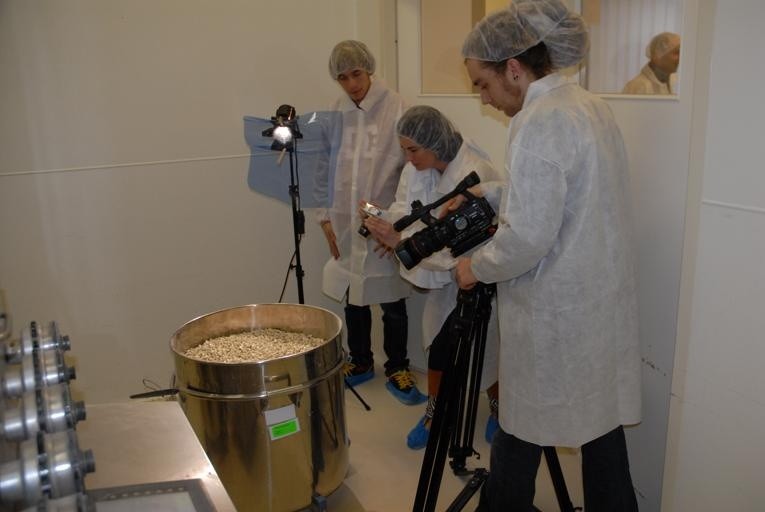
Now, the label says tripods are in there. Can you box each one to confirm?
[411,288,578,512]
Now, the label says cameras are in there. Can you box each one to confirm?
[358,203,382,238]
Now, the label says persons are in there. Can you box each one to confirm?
[358,105,502,449]
[622,32,680,95]
[313,40,420,405]
[440,10,641,512]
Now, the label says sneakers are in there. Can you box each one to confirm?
[385,369,421,405]
[344,358,374,389]
[407,406,434,449]
[485,400,499,443]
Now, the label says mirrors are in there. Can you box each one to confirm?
[414,0,519,99]
[573,0,686,101]
